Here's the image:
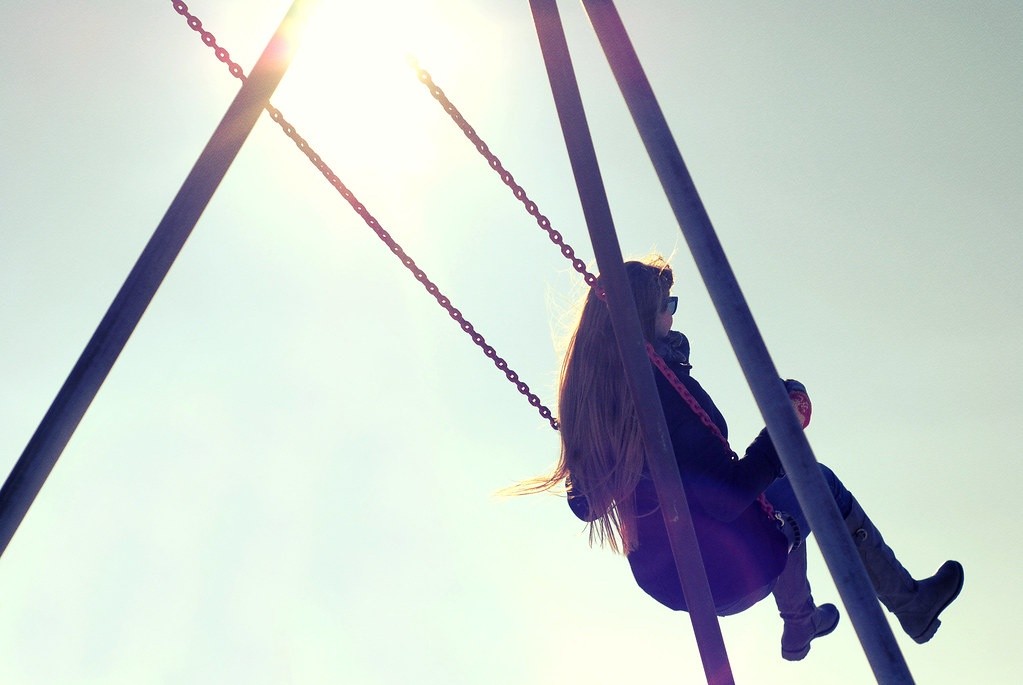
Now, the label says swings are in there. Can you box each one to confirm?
[169,0,803,617]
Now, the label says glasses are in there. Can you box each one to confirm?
[655,297,678,314]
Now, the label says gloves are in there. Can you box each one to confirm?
[780,378,811,430]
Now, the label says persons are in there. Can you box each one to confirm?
[489,261,965,661]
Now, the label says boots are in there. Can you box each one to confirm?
[773,535,840,661]
[845,496,964,645]
